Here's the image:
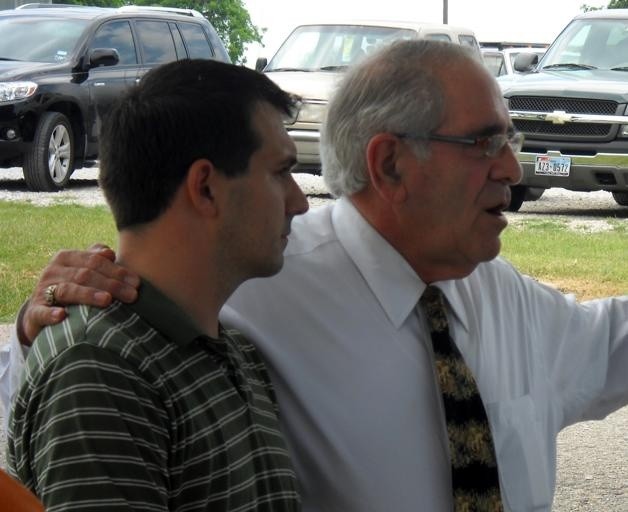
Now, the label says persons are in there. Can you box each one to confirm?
[15,39,628,507]
[6,57,309,511]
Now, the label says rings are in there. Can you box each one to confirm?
[41,279,60,307]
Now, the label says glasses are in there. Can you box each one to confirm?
[396,131,525,159]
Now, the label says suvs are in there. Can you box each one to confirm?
[0,0,236,193]
[495,8,628,209]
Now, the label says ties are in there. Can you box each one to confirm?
[420,285,504,512]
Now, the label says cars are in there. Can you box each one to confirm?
[254,21,484,172]
[480,44,580,77]
